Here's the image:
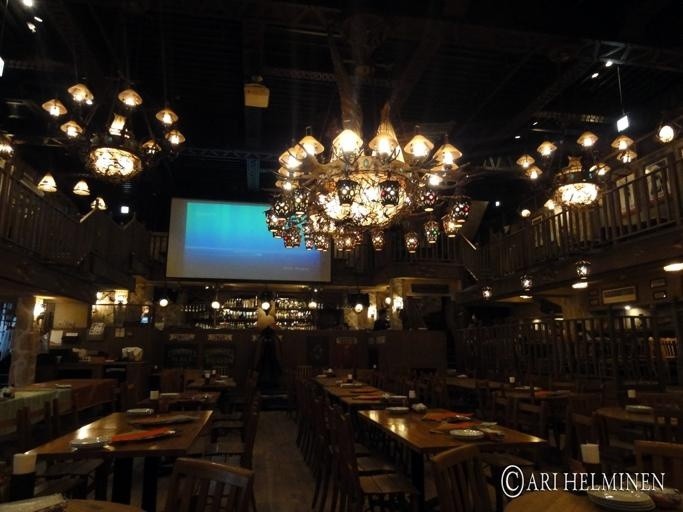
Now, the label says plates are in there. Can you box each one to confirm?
[517,386,542,391]
[69,439,111,449]
[625,405,654,414]
[316,367,426,416]
[585,486,657,512]
[126,408,153,415]
[160,392,180,398]
[56,385,71,388]
[447,428,484,442]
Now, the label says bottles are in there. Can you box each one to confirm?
[7,472,37,500]
[204,373,216,384]
[627,398,637,405]
[170,335,234,364]
[2,384,16,398]
[579,460,605,489]
[182,296,260,329]
[276,298,316,330]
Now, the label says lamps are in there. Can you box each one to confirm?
[36,79,186,214]
[0,133,16,160]
[516,112,675,212]
[575,259,591,283]
[481,282,494,301]
[267,61,489,255]
[520,272,536,293]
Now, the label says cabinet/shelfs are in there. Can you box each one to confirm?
[152,286,319,328]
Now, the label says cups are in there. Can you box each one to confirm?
[158,398,168,412]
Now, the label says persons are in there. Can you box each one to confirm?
[650,165,663,195]
[589,319,612,362]
[373,309,390,331]
[400,309,415,332]
